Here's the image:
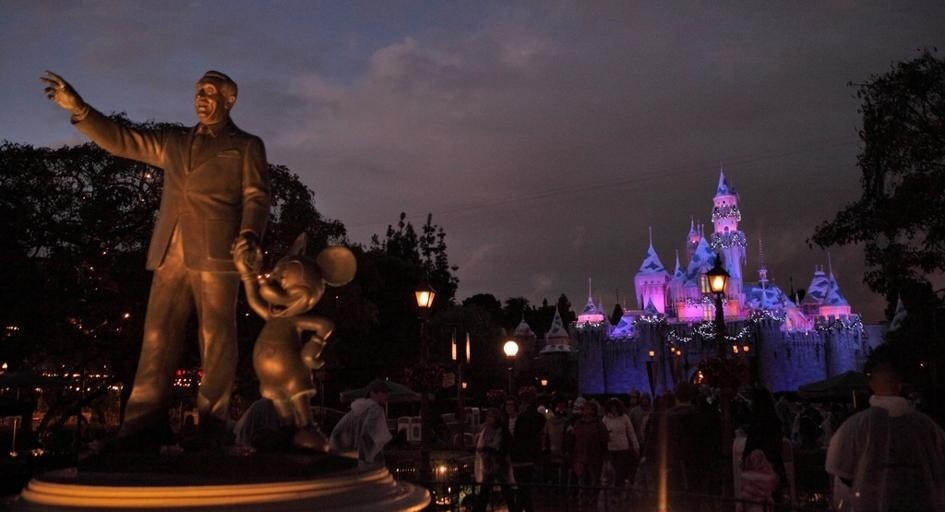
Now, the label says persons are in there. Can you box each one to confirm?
[731,367,940,512]
[473,373,676,512]
[333,380,393,470]
[40,70,271,461]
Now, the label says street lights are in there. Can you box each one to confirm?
[649,346,683,512]
[414,270,437,489]
[503,340,519,397]
[705,252,734,497]
[733,344,750,362]
[541,377,548,393]
